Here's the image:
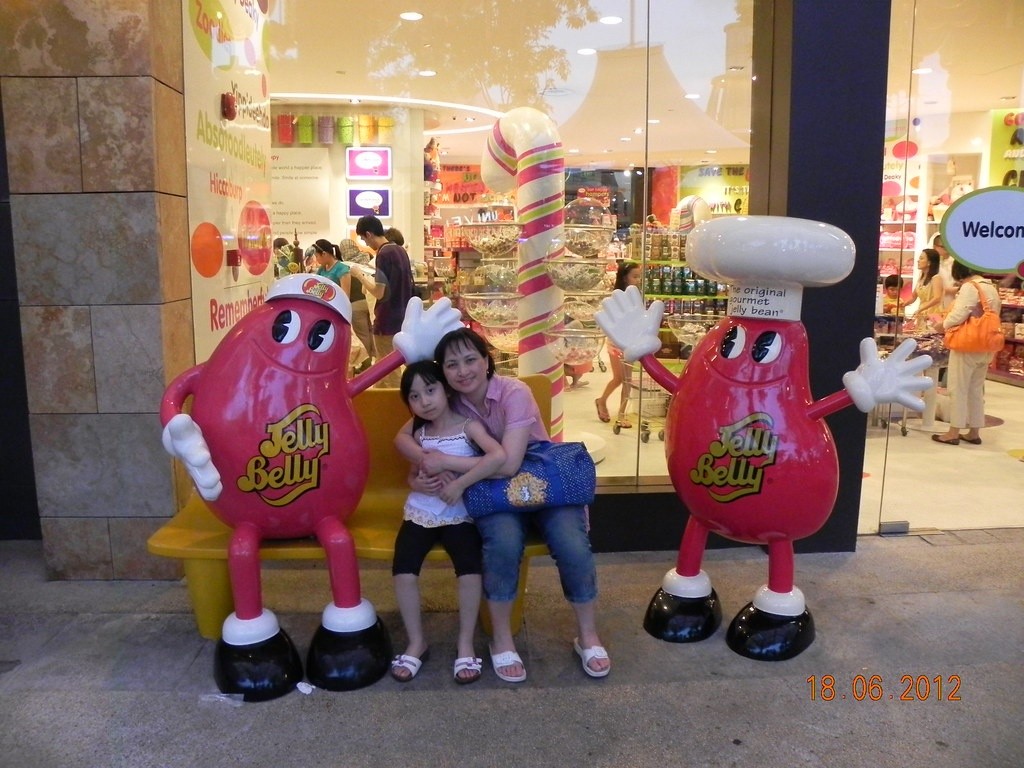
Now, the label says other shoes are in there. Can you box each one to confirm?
[959,434,981,444]
[354,364,372,375]
[932,434,959,445]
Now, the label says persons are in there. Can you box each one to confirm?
[883,235,961,382]
[274,214,416,374]
[595,262,641,428]
[391,328,611,683]
[934,259,1001,445]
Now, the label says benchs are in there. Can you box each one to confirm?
[148,374,555,640]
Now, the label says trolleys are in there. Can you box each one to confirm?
[620,355,666,443]
[872,402,912,437]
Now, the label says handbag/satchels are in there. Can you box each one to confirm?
[944,280,1004,351]
[413,285,429,300]
[462,440,596,519]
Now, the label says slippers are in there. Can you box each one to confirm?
[489,640,526,682]
[574,637,610,677]
[390,648,430,682]
[453,651,482,685]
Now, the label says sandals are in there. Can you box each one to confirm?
[621,421,632,428]
[595,398,610,422]
